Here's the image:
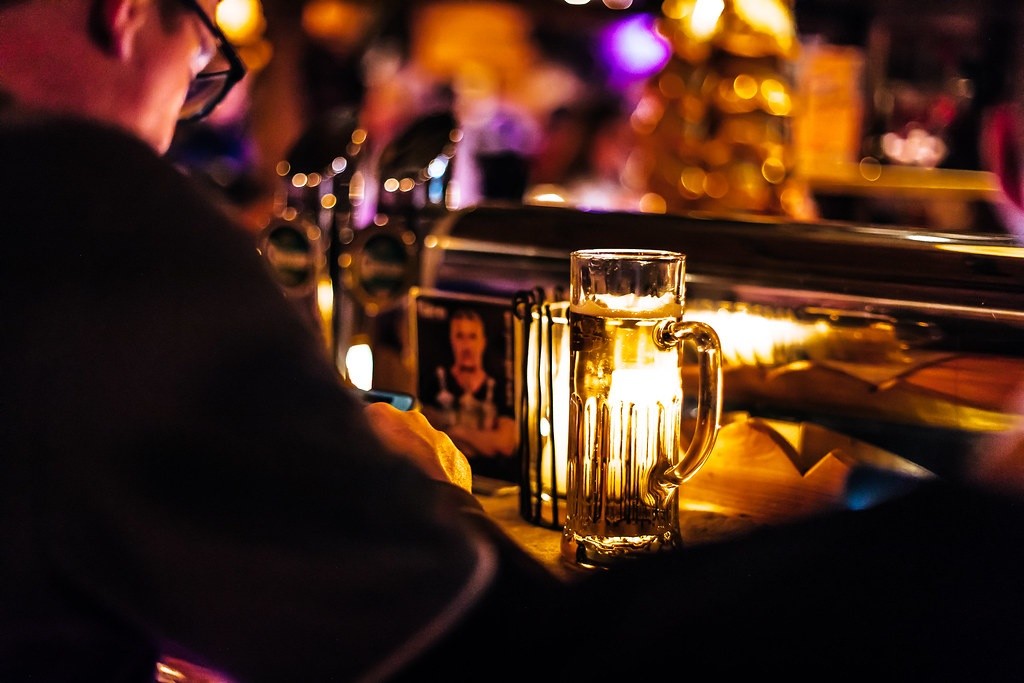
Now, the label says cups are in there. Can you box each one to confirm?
[559,249,725,573]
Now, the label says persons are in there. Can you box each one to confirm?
[417,308,517,461]
[1,1,608,683]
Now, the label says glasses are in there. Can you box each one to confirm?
[176,0,248,126]
